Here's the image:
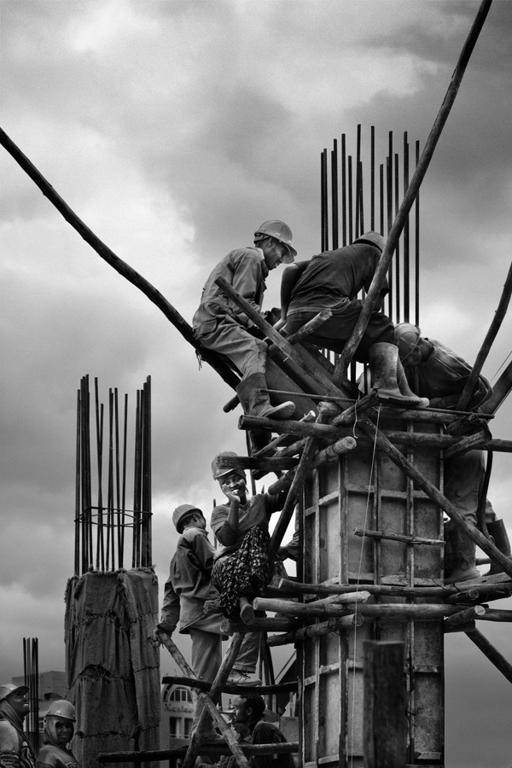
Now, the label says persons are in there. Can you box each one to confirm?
[191,218,296,478]
[34,698,82,768]
[0,682,37,768]
[272,229,430,409]
[231,712,252,759]
[392,320,512,584]
[206,449,290,616]
[233,693,296,768]
[149,502,264,746]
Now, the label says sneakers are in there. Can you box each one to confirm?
[194,733,227,746]
[225,669,262,687]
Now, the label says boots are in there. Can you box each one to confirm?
[249,428,278,480]
[397,352,430,409]
[234,371,296,438]
[444,523,480,584]
[367,342,423,405]
[481,518,512,577]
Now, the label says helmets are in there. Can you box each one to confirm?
[211,450,241,479]
[173,503,203,534]
[254,219,297,257]
[393,322,420,361]
[46,699,76,722]
[0,683,30,701]
[353,231,386,252]
